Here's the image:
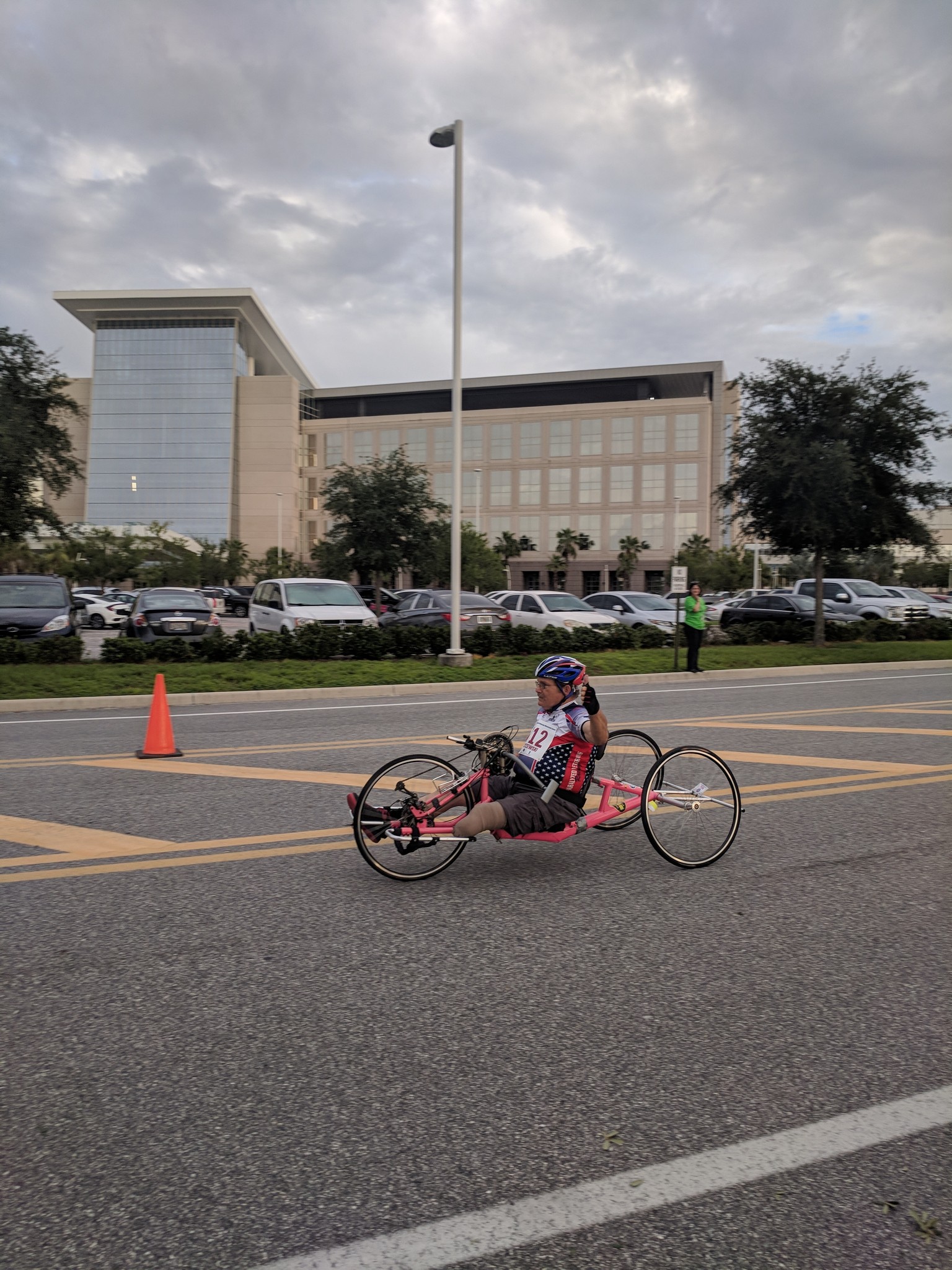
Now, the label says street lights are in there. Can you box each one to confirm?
[428,117,476,669]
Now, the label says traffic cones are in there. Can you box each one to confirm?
[134,673,185,760]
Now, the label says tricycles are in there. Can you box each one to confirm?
[342,726,746,883]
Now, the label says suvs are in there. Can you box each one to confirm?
[247,577,380,639]
[791,578,932,627]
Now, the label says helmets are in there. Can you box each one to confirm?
[534,655,587,686]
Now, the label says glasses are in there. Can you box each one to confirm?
[535,681,557,689]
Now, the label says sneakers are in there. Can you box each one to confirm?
[347,793,393,843]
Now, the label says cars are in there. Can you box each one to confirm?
[0,571,86,640]
[492,590,624,636]
[880,586,952,620]
[71,586,149,606]
[719,593,868,628]
[147,587,227,616]
[375,590,511,637]
[581,590,687,639]
[202,586,251,618]
[483,591,521,602]
[661,588,794,609]
[116,590,221,646]
[352,584,404,617]
[387,587,445,599]
[72,594,134,630]
[927,594,952,605]
[704,598,748,622]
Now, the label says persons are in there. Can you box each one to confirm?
[683,581,706,673]
[346,656,608,838]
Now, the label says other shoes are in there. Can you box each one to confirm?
[689,669,705,673]
